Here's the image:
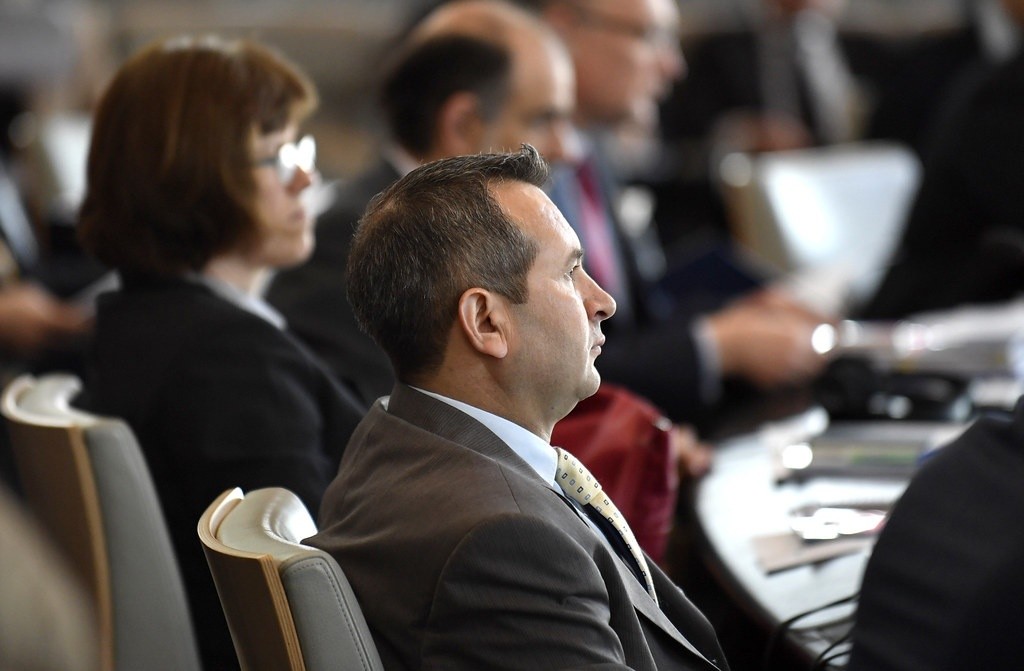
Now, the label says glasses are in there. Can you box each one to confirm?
[244,132,317,186]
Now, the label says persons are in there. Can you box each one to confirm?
[285,0,715,574]
[844,393,1024,671]
[65,34,377,670]
[691,0,884,181]
[300,142,756,671]
[0,13,127,389]
[523,0,864,443]
[877,1,1024,323]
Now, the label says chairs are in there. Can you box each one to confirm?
[0,371,203,671]
[197,484,387,671]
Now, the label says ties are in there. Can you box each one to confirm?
[554,447,661,611]
[578,151,628,307]
